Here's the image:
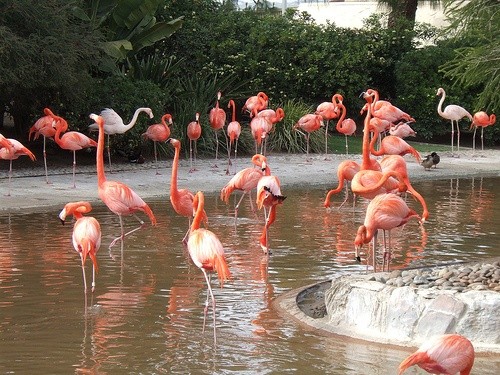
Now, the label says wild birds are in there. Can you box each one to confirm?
[1,108,154,197]
[470,111,496,158]
[353,194,424,262]
[165,138,208,232]
[89,112,157,250]
[399,334,474,375]
[186,191,234,308]
[58,201,102,294]
[436,87,473,157]
[219,153,287,255]
[323,87,439,225]
[141,92,356,177]
